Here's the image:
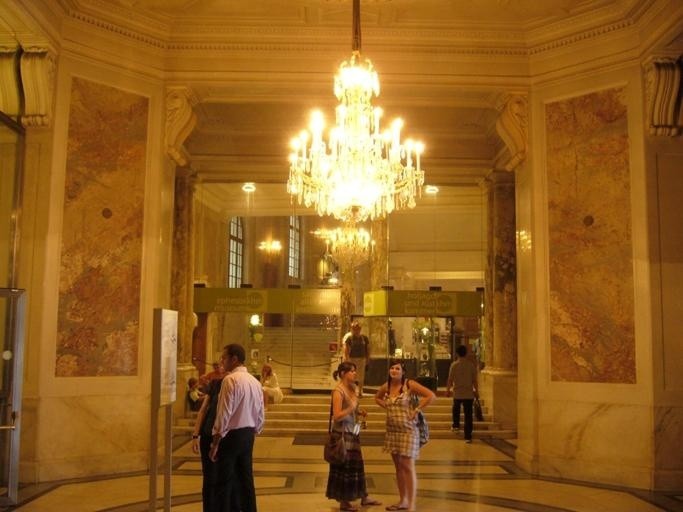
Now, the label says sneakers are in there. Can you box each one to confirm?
[450,426,459,434]
[465,439,471,443]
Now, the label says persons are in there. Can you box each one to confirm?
[192,354,229,512]
[207,343,265,512]
[375,358,437,512]
[258,364,284,410]
[445,345,480,443]
[200,361,225,382]
[341,320,369,397]
[325,361,382,511]
[188,379,208,411]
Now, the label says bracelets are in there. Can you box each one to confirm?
[192,436,198,439]
[210,442,219,449]
[415,408,421,413]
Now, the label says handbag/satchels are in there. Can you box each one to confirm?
[413,394,428,444]
[474,396,485,421]
[323,437,346,465]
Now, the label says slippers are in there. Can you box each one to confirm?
[385,504,408,510]
[360,499,382,505]
[339,505,358,511]
